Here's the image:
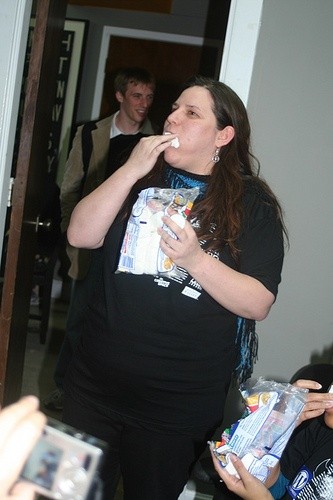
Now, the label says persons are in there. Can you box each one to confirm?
[54,77,284,500]
[210,378,333,500]
[0,394,47,500]
[42,65,156,411]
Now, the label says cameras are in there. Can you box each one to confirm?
[17,413,107,500]
